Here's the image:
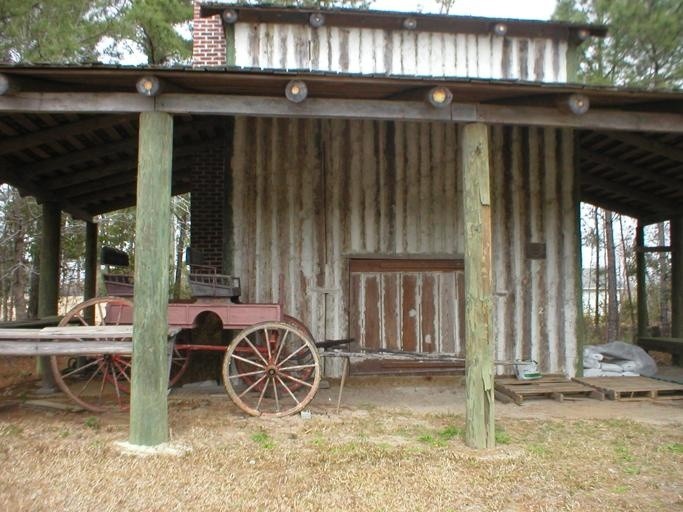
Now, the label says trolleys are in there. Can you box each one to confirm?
[1,246,354,422]
